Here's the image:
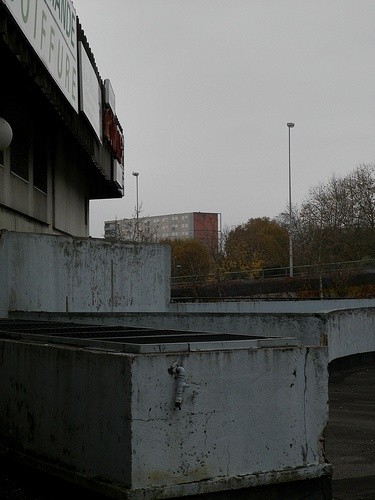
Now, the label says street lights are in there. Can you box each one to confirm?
[133,172,139,231]
[287,122,296,278]
